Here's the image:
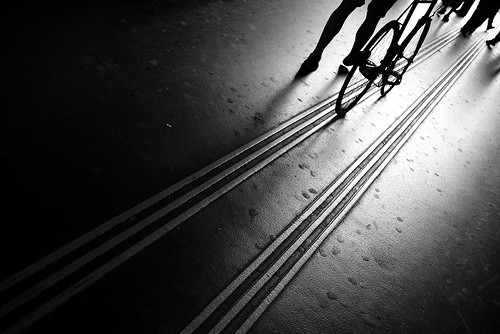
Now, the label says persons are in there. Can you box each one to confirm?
[486,31,500,50]
[460,0,500,35]
[437,0,467,22]
[300,0,397,70]
[486,3,500,30]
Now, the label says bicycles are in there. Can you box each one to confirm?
[335,0,438,115]
